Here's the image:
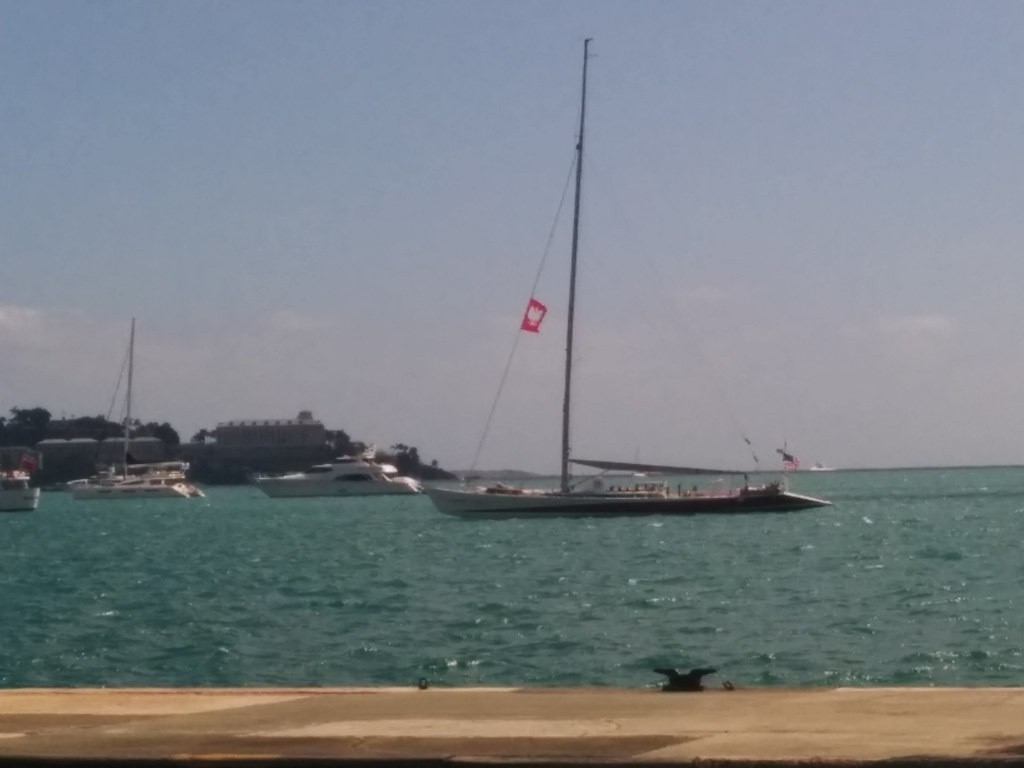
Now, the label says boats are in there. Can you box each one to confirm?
[0,453,43,511]
[254,442,424,499]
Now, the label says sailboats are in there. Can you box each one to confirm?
[419,31,838,515]
[67,314,206,499]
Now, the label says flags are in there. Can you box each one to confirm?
[520,298,547,334]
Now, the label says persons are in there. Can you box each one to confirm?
[606,473,752,498]
